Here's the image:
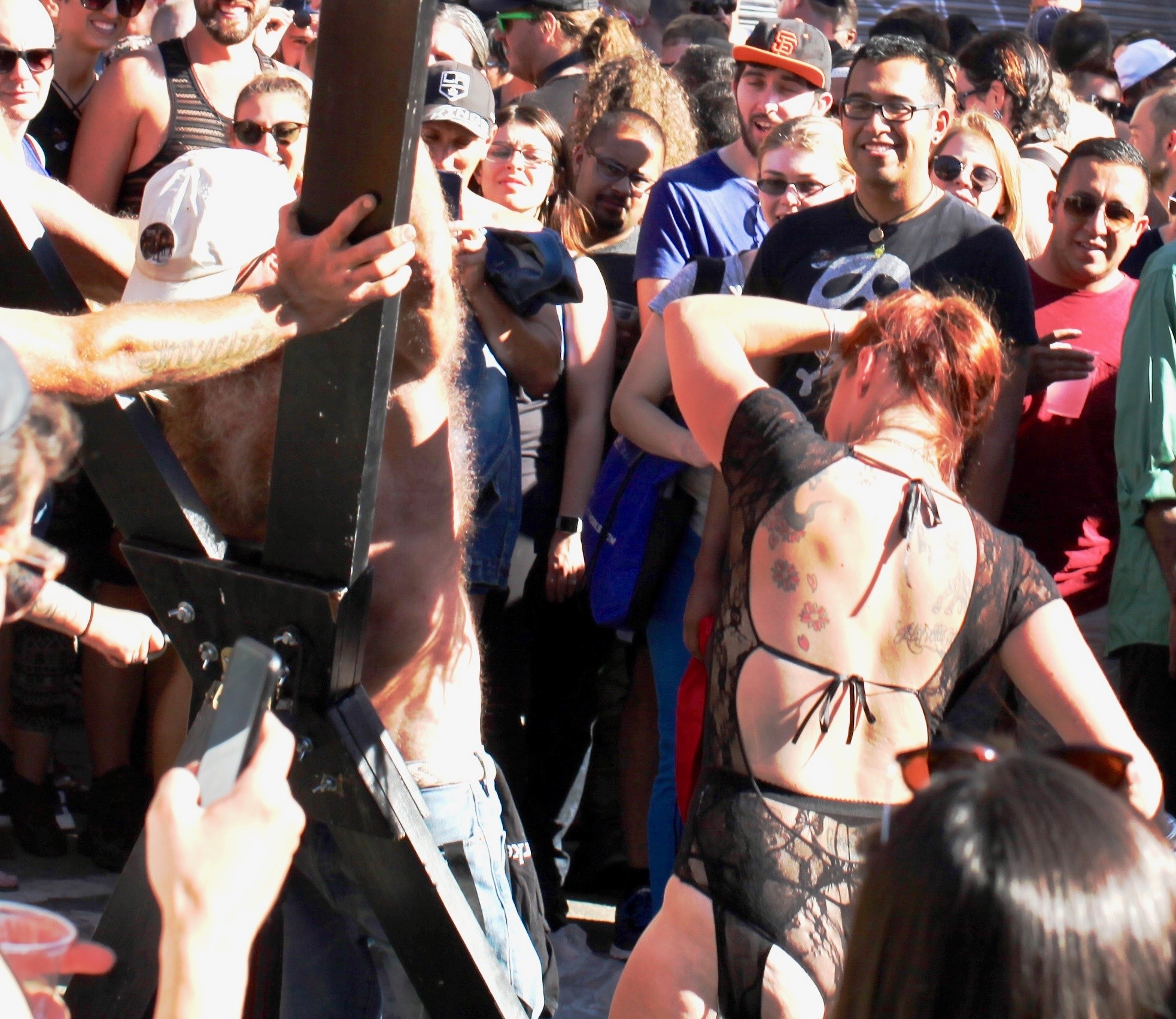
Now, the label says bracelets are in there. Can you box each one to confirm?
[73,601,94,655]
[813,304,835,362]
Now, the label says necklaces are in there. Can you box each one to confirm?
[854,185,934,243]
[184,36,235,149]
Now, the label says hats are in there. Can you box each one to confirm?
[421,61,495,140]
[1113,39,1176,92]
[118,149,297,308]
[733,17,832,90]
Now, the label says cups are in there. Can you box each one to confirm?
[0,899,79,1019]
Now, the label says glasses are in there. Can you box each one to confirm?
[952,88,1011,114]
[1087,95,1126,116]
[486,142,554,168]
[585,142,659,192]
[758,175,841,196]
[232,119,308,144]
[497,11,576,33]
[932,155,1004,192]
[282,0,319,14]
[841,98,941,120]
[1167,192,1176,216]
[1058,191,1142,230]
[0,48,55,74]
[81,0,147,18]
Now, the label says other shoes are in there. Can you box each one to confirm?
[610,886,651,964]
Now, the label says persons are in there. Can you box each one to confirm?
[825,754,1176,1019]
[0,0,1176,969]
[0,340,307,1019]
[117,137,563,1019]
[606,286,1162,1019]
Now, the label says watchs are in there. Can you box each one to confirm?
[554,516,583,535]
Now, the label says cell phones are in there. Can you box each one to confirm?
[193,633,283,808]
